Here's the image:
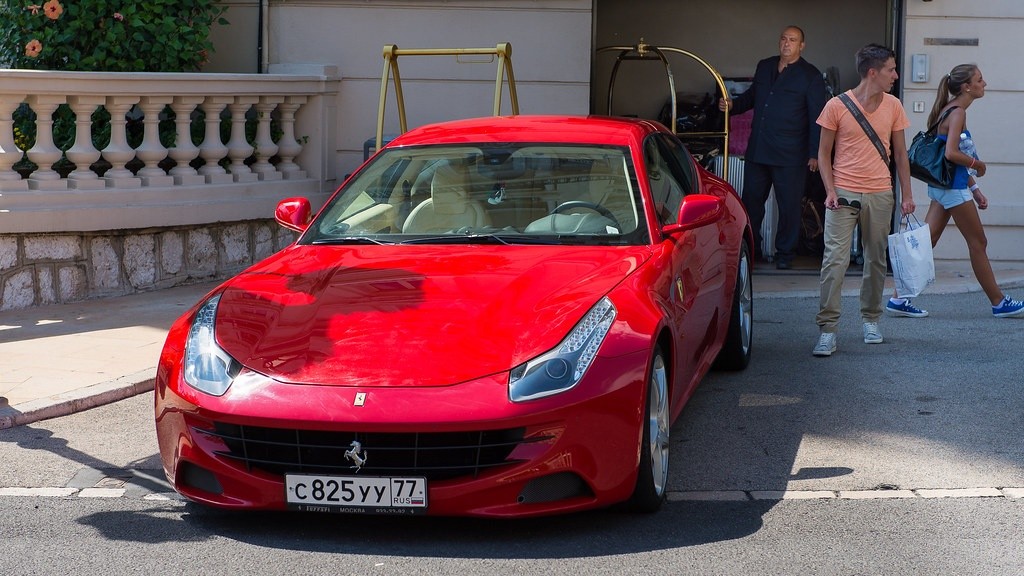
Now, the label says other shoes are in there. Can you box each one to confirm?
[748,257,763,270]
[775,257,793,270]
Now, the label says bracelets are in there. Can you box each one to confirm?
[970,158,976,168]
[968,183,979,192]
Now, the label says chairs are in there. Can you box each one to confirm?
[406,152,680,236]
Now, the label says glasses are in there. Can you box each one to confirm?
[832,198,862,216]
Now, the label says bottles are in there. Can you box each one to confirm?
[959,133,980,176]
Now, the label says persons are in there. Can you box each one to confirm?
[811,40,917,357]
[717,25,829,269]
[886,68,1022,318]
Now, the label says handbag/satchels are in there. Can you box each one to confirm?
[889,211,935,296]
[908,106,966,191]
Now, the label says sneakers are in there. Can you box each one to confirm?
[992,295,1024,317]
[862,322,883,344]
[812,332,838,355]
[886,299,928,318]
